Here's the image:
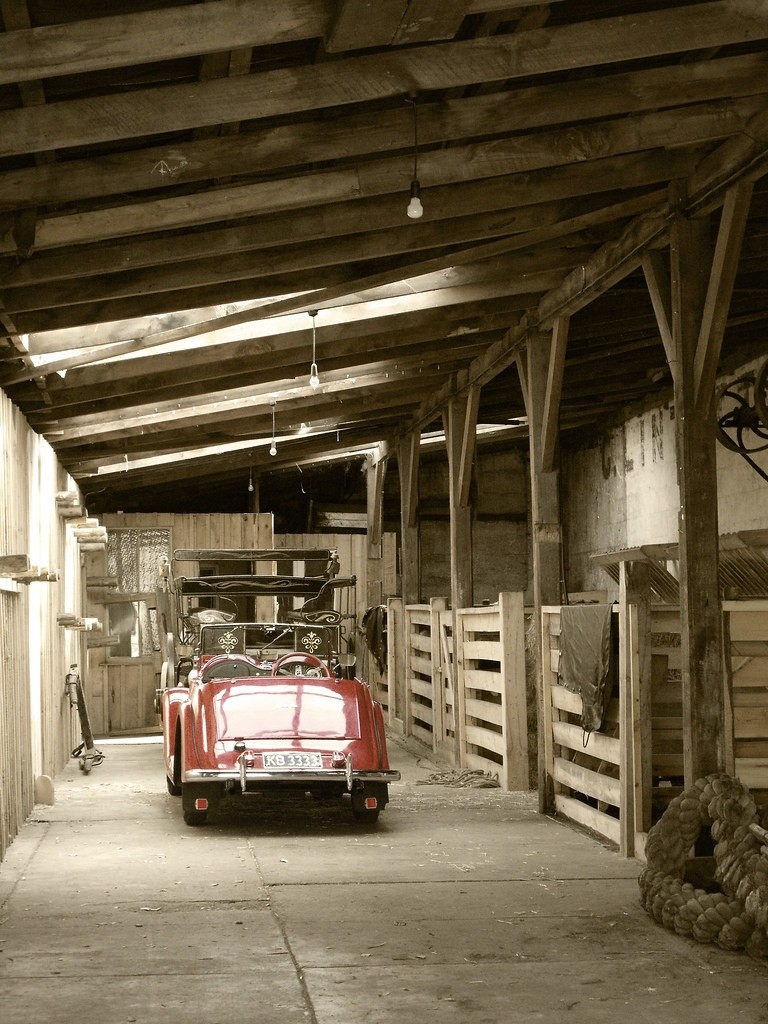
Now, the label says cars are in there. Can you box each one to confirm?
[155,620,402,825]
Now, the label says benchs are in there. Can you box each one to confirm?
[287,609,354,651]
[200,655,333,679]
[183,605,237,649]
